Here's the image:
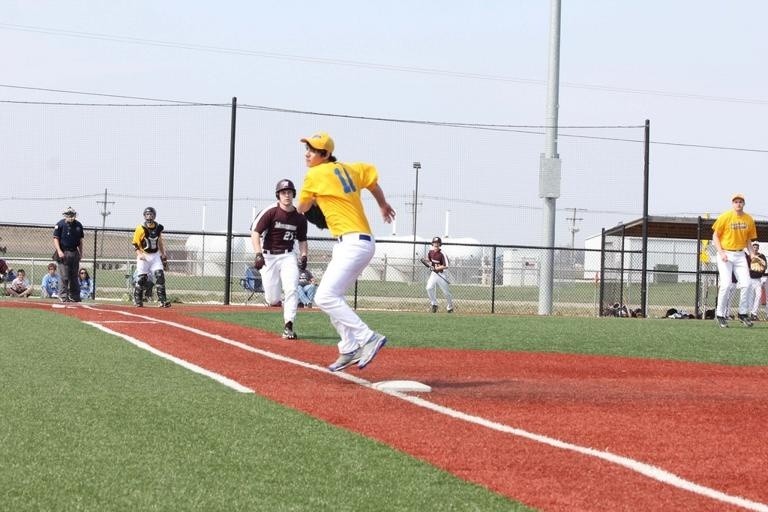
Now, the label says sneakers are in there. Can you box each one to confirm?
[750,314,758,321]
[307,301,312,307]
[59,296,69,303]
[70,295,81,302]
[328,349,363,372]
[447,306,453,312]
[717,316,729,328]
[136,299,141,306]
[737,315,752,327]
[282,327,297,340]
[160,300,170,308]
[432,304,438,312]
[357,331,386,370]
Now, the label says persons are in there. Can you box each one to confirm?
[131,207,171,308]
[298,269,317,308]
[77,268,94,299]
[9,269,34,298]
[0,259,15,283]
[52,207,85,303]
[249,178,309,340]
[711,193,758,329]
[42,263,60,298]
[749,244,767,321]
[426,236,454,313]
[297,132,396,372]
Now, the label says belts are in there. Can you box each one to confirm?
[728,250,743,252]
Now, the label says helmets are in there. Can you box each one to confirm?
[275,179,296,193]
[143,207,155,219]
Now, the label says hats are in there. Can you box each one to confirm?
[431,237,441,244]
[301,131,334,152]
[731,193,745,202]
